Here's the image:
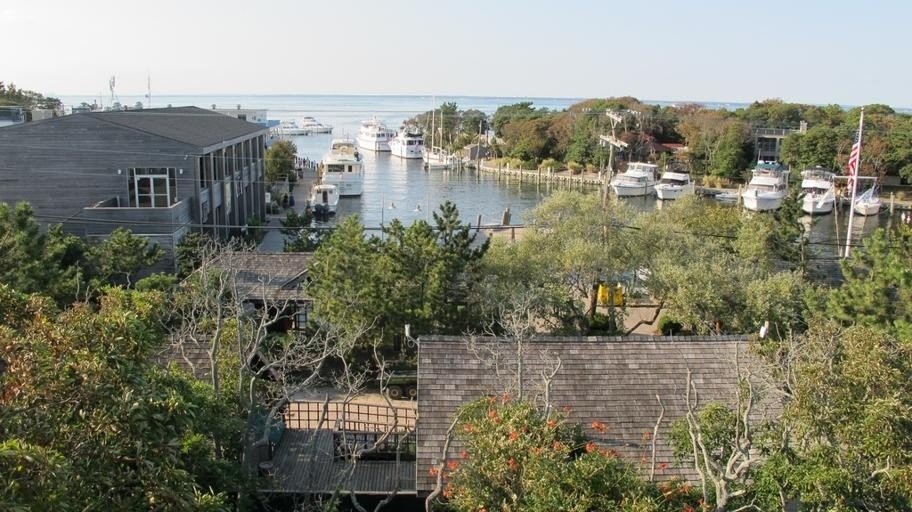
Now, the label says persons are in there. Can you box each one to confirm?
[388,203,395,209]
[283,193,294,206]
[593,268,602,290]
[298,157,317,167]
[305,202,329,221]
[415,205,421,211]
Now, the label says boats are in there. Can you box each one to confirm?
[612,160,879,217]
[270,117,465,215]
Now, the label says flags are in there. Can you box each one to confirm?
[847,125,863,196]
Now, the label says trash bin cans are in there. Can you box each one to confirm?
[258,462,273,488]
[289,195,295,206]
[300,172,303,178]
